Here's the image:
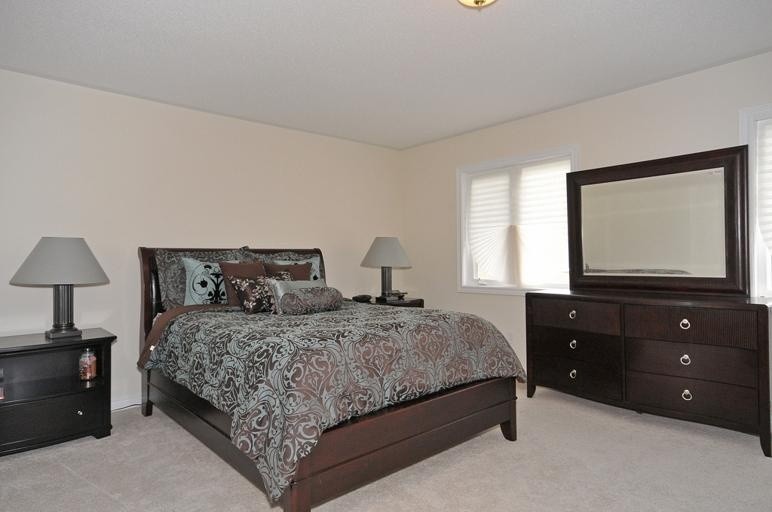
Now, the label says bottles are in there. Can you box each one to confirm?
[79,347,97,380]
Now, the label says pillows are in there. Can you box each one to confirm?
[220,261,266,306]
[281,288,343,312]
[181,257,240,307]
[231,249,325,281]
[153,247,249,313]
[228,271,294,315]
[267,255,322,282]
[261,277,327,314]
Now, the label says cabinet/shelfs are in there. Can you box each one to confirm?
[526,292,769,454]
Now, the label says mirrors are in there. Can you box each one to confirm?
[566,145,750,293]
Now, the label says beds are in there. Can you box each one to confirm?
[139,252,518,512]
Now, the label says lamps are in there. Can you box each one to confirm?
[8,237,110,340]
[457,0,499,13]
[358,238,412,299]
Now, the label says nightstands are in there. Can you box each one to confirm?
[0,328,116,458]
[353,297,424,308]
